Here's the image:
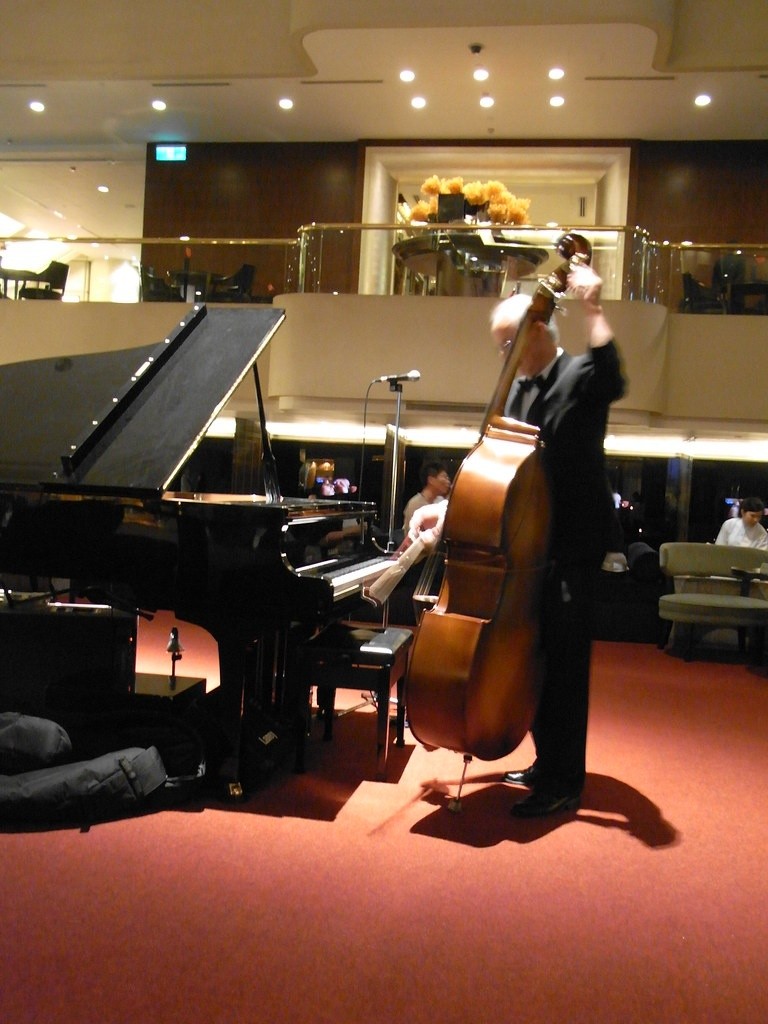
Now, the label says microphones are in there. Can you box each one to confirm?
[375,370,421,383]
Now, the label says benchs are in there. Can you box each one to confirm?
[659,542,768,656]
[299,623,415,777]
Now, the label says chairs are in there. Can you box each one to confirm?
[712,254,763,312]
[682,272,728,316]
[21,261,69,301]
[212,262,256,302]
[141,265,172,302]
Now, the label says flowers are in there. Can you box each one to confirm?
[398,176,531,236]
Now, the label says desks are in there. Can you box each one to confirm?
[0,269,38,300]
[166,269,220,302]
[721,280,768,315]
[392,233,549,298]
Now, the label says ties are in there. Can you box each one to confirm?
[508,379,534,420]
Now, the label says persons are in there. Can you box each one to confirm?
[395,461,452,592]
[714,495,768,555]
[482,253,633,821]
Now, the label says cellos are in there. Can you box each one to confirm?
[403,231,594,814]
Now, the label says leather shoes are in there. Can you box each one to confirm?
[510,792,580,817]
[503,766,537,785]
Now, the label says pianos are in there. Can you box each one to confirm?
[1,302,405,806]
